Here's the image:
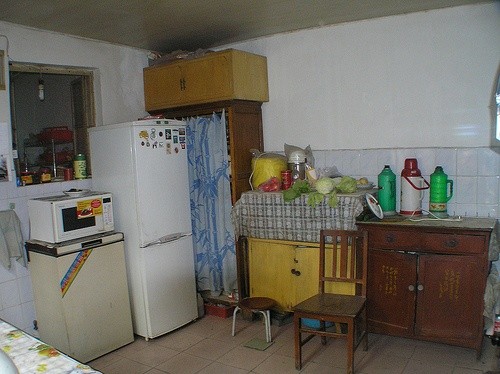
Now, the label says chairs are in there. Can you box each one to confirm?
[292,230,368,374]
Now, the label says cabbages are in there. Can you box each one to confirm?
[284,176,357,201]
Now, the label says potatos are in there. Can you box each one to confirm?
[356,177,368,184]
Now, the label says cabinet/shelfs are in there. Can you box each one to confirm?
[143,48,269,112]
[24,138,75,177]
[357,215,497,360]
[161,104,264,315]
[230,190,378,316]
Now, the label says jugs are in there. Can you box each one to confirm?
[399,158,430,216]
[429,165,453,219]
[377,165,398,217]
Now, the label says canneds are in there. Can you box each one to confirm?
[20,167,53,185]
[63,168,73,180]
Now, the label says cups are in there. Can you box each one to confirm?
[288,161,305,182]
[281,170,292,190]
[64,168,73,180]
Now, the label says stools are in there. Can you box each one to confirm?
[232,297,274,342]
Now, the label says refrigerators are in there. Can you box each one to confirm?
[85,118,199,340]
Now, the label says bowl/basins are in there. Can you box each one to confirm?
[63,189,90,197]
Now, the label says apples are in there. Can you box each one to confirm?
[259,176,280,191]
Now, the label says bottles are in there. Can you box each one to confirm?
[491,311,500,346]
[73,153,87,179]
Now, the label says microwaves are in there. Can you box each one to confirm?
[28,191,115,244]
